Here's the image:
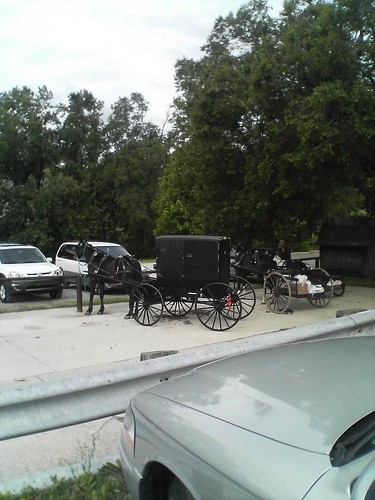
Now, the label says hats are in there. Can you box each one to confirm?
[277,240,286,246]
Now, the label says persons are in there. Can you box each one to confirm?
[278,239,290,261]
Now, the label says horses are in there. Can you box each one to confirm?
[76,236,143,320]
[235,242,278,305]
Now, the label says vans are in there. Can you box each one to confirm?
[56,241,130,293]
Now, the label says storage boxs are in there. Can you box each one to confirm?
[288,283,308,295]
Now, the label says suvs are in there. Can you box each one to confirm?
[1,243,65,303]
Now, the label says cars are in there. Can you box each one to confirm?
[119,331,375,499]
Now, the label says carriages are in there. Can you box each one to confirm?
[230,242,334,315]
[75,232,257,333]
